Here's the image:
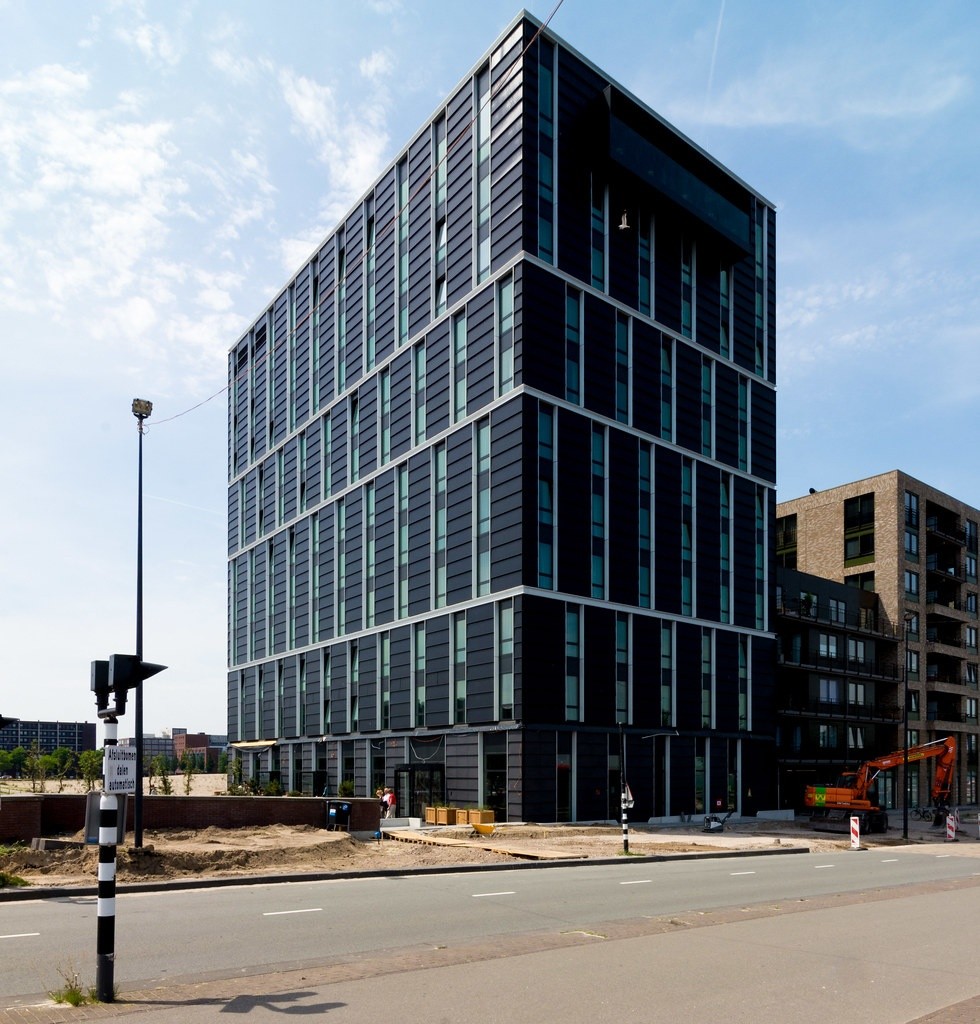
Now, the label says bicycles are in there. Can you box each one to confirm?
[910,804,933,822]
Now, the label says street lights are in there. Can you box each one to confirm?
[902,611,921,839]
[131,397,153,850]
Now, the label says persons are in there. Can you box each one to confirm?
[376,789,385,818]
[382,788,390,814]
[386,789,396,818]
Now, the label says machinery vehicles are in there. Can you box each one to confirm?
[804,736,956,836]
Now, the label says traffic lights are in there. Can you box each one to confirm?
[108,654,169,689]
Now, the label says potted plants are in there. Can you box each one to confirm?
[425,800,495,825]
